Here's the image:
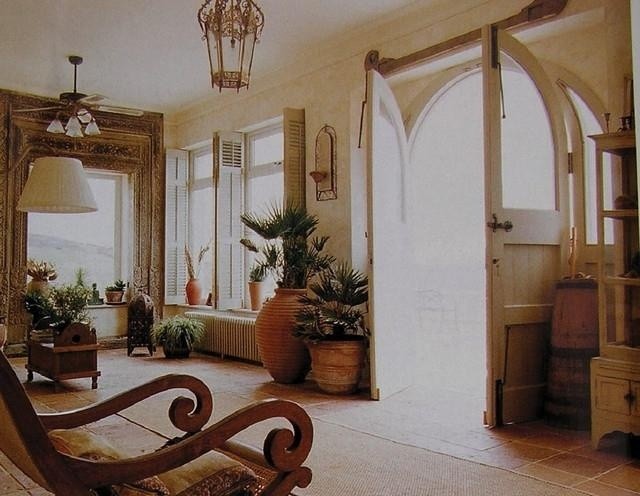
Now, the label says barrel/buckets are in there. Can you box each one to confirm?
[551,277,598,349]
[541,351,590,430]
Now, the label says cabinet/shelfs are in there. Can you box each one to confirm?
[584,126,640,452]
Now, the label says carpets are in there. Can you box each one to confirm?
[113,388,599,496]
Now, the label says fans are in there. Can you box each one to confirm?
[10,54,145,118]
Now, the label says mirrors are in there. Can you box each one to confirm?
[311,122,338,201]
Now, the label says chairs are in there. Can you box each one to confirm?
[0,321,316,496]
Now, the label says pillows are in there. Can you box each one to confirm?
[44,428,171,496]
[155,444,261,496]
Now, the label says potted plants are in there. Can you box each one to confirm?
[286,254,373,397]
[19,255,58,304]
[247,262,270,311]
[237,192,339,387]
[147,312,209,360]
[104,279,126,304]
[47,281,96,345]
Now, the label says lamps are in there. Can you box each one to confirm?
[0,141,100,215]
[45,102,102,138]
[197,0,266,93]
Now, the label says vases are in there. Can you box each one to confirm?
[184,278,201,306]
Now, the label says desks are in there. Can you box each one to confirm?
[24,315,102,395]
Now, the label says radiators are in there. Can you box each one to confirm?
[180,309,265,365]
